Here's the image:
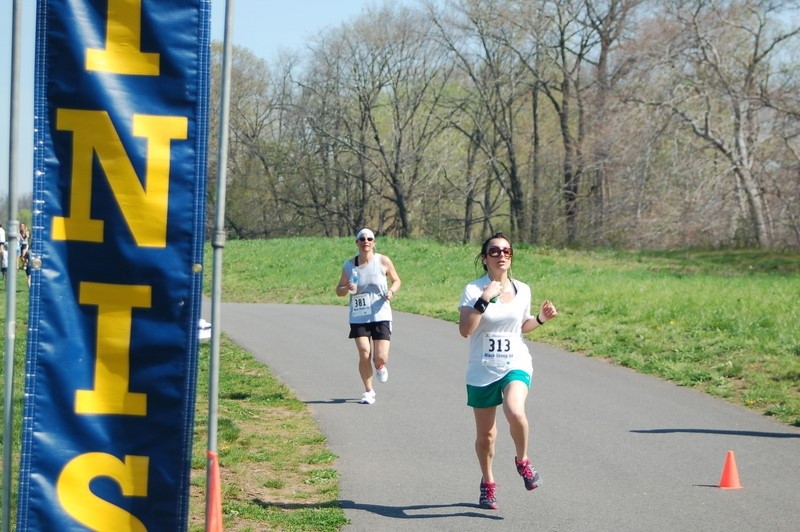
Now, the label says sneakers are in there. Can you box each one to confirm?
[361,389,376,404]
[515,456,541,491]
[479,476,497,509]
[376,364,388,382]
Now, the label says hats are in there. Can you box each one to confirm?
[355,229,376,244]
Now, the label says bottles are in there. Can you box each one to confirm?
[348,271,358,294]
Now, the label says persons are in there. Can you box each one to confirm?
[0,223,31,288]
[336,227,401,405]
[458,234,557,508]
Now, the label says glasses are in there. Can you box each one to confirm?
[359,237,373,242]
[485,248,513,258]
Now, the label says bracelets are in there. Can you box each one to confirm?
[536,313,544,325]
[389,289,394,295]
[474,297,489,313]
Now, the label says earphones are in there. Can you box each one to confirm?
[484,258,487,261]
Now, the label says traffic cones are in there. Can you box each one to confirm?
[718,451,743,490]
